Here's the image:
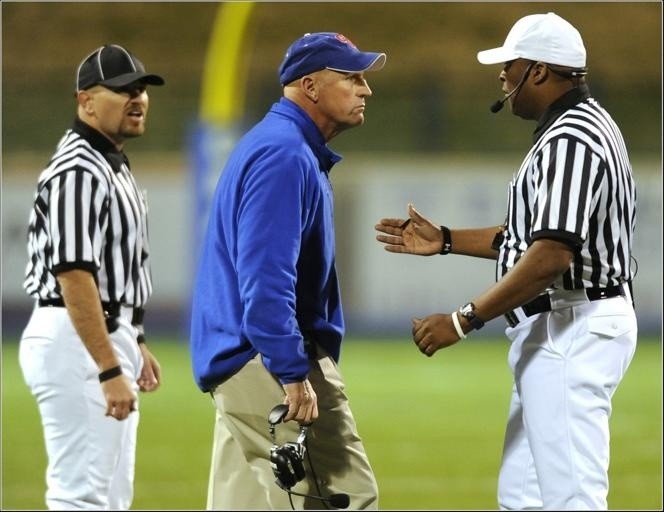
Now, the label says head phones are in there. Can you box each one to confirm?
[268,404,309,488]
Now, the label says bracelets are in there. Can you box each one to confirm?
[97,363,123,383]
[440,226,450,256]
[452,310,467,341]
[135,334,148,344]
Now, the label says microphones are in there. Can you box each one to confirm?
[490,62,588,113]
[275,479,350,508]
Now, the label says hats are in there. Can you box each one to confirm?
[476,12,587,69]
[278,31,386,86]
[76,43,165,91]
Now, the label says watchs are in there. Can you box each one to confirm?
[459,302,483,330]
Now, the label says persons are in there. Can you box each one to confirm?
[189,32,390,510]
[373,12,640,511]
[12,41,163,510]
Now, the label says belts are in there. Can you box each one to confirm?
[504,285,624,328]
[36,297,145,324]
